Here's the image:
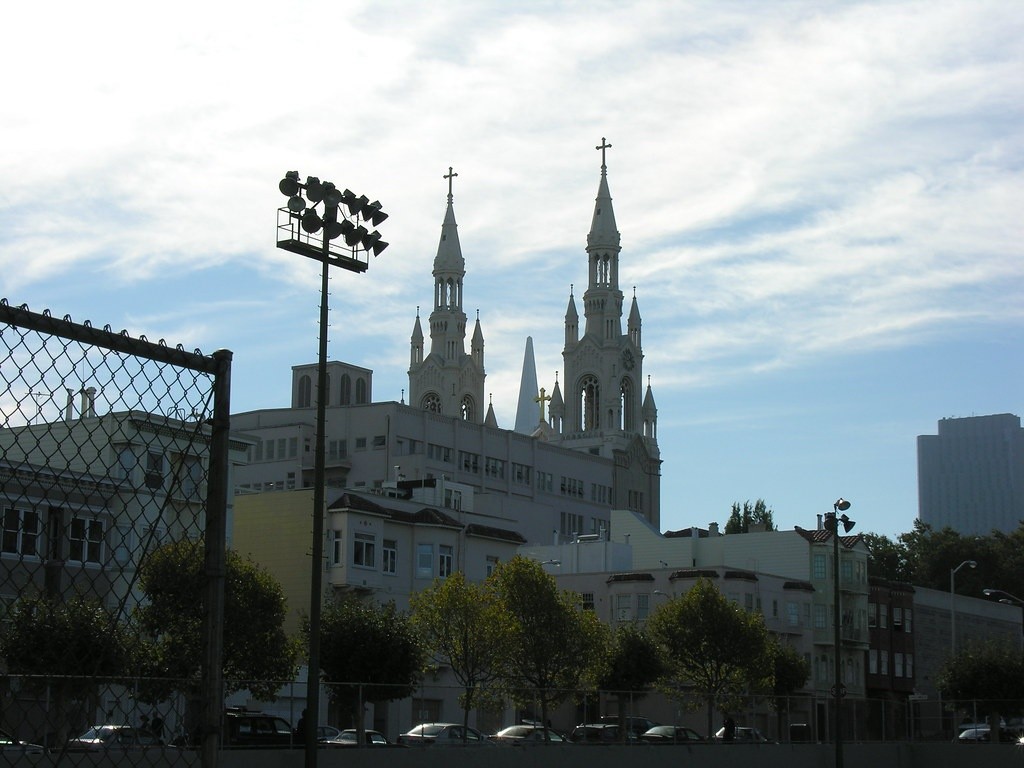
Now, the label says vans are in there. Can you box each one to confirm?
[600,714,660,741]
[225,710,302,749]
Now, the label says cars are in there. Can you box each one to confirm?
[488,723,574,744]
[568,722,625,744]
[318,724,341,748]
[323,728,398,748]
[0,740,47,753]
[958,728,1012,743]
[705,726,780,744]
[65,724,167,751]
[637,725,707,742]
[396,722,498,746]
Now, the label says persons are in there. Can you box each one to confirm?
[297,709,307,744]
[150,711,165,738]
[722,712,735,745]
[139,715,150,729]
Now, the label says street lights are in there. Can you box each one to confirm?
[275,169,391,768]
[826,497,856,768]
[950,560,979,741]
